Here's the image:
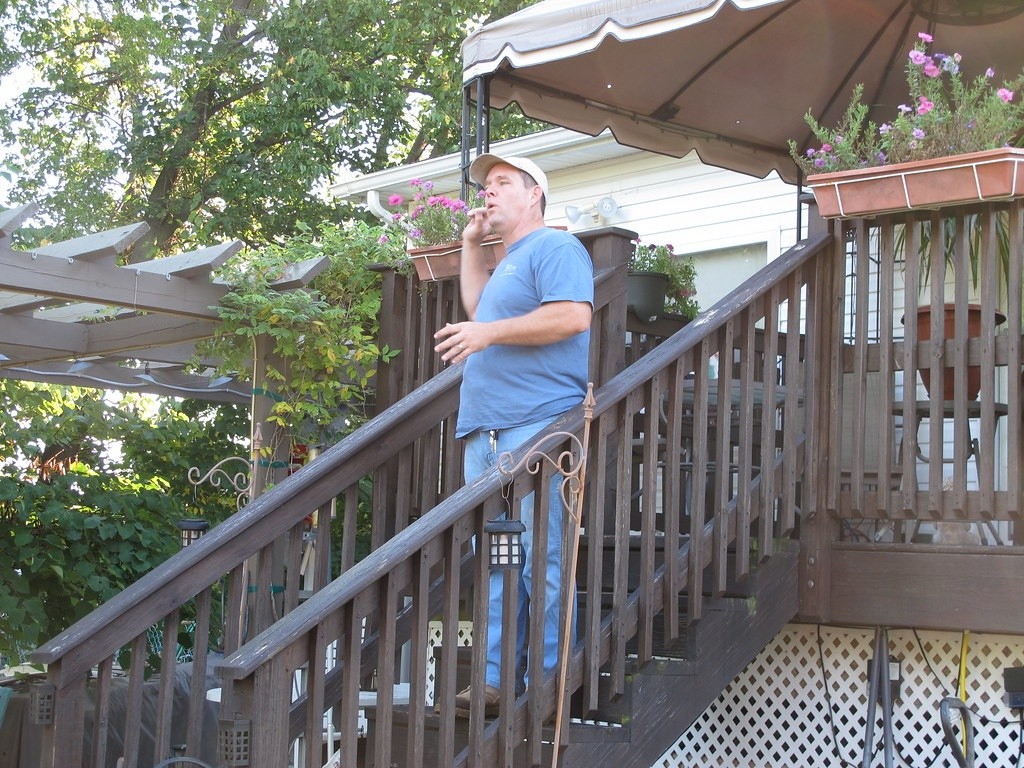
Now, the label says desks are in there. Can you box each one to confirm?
[874,400,1008,546]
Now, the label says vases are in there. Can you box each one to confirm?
[901,304,1006,401]
[627,271,669,324]
[407,226,568,281]
[806,147,1024,221]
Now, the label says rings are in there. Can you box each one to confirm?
[459,344,463,352]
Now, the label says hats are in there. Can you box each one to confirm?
[470,153,548,205]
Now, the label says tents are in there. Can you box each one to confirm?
[460,0,1024,244]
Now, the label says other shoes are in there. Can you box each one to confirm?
[434,683,516,718]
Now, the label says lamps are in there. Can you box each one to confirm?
[483,471,526,570]
[177,480,210,550]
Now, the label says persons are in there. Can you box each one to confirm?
[434,153,593,718]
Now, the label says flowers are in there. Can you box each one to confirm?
[786,32,1024,176]
[627,237,701,320]
[376,178,487,276]
